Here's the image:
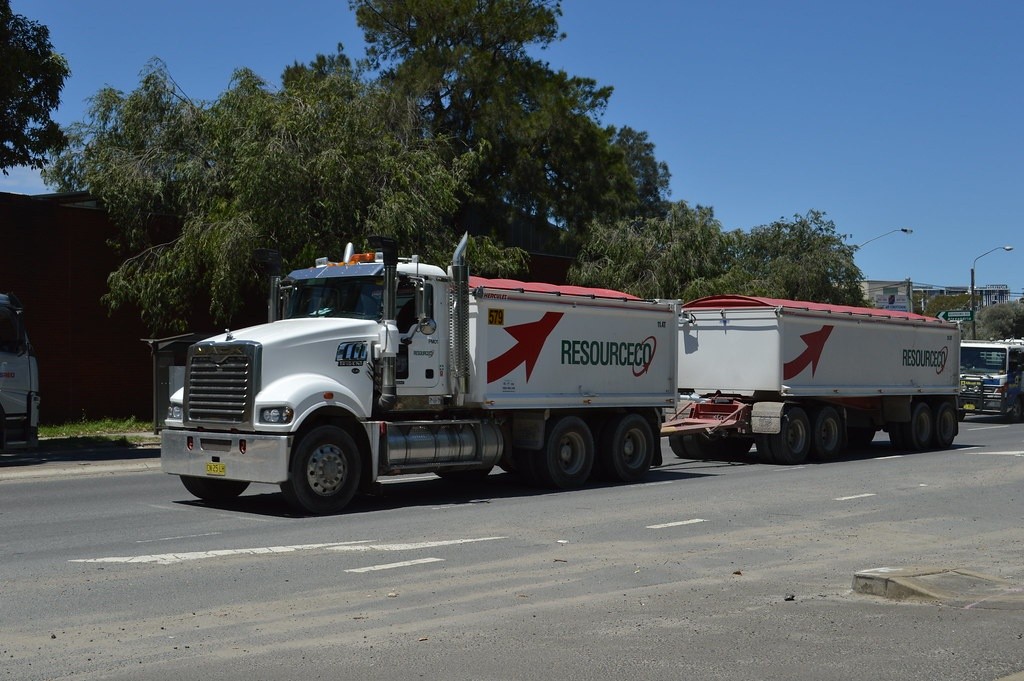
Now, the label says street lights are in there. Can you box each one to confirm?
[970,245,1014,336]
[854,228,914,258]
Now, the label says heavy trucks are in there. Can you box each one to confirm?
[960,338,1024,426]
[159,232,683,517]
[661,291,968,468]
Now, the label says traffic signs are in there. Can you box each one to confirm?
[935,310,973,322]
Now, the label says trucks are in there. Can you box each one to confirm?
[0,291,41,465]
